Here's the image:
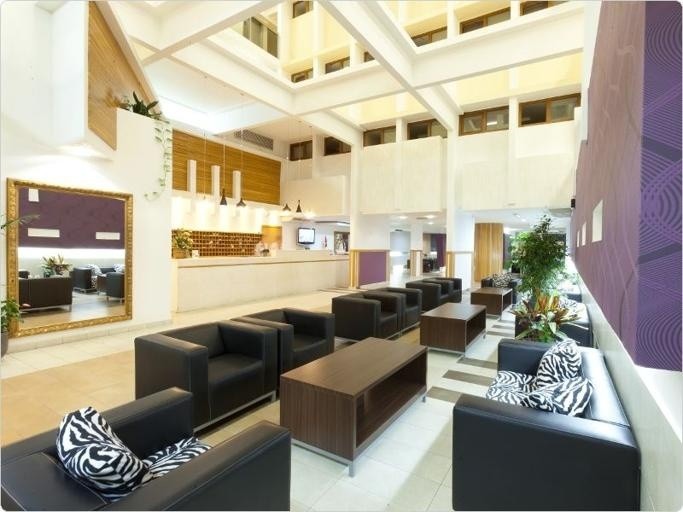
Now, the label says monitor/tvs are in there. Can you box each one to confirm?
[298,228,315,244]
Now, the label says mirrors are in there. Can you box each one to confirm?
[6,176,132,340]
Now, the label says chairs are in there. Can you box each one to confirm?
[1,385,291,511]
[481,276,522,305]
[404,279,450,310]
[18,265,124,313]
[368,287,422,337]
[426,277,462,310]
[515,303,593,347]
[558,281,581,302]
[134,318,279,437]
[453,338,641,510]
[330,292,401,341]
[230,307,335,373]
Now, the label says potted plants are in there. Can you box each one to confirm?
[171,228,193,259]
[125,90,173,201]
[0,299,21,356]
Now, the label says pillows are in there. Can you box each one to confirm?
[55,406,152,503]
[492,272,511,288]
[521,376,595,417]
[537,341,582,381]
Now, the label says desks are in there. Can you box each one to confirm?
[471,287,513,319]
[419,302,487,356]
[279,337,429,477]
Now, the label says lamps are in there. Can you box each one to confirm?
[282,119,292,213]
[217,130,229,206]
[198,129,212,208]
[295,121,303,213]
[235,128,247,207]
